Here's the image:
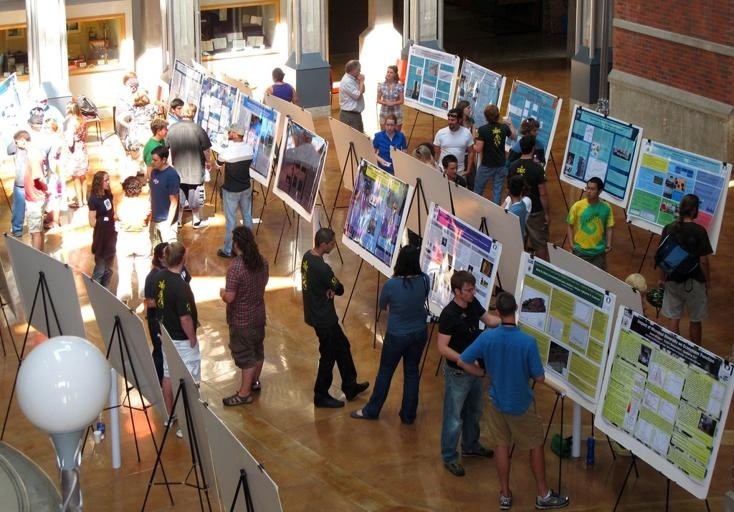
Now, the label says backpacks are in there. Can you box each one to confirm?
[654,223,700,284]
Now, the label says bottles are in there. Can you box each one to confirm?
[587,436,596,465]
[97,413,105,440]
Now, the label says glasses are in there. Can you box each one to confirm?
[584,186,598,191]
[526,117,535,124]
[447,113,459,119]
[459,286,477,292]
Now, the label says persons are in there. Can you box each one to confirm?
[213,123,256,257]
[141,118,168,203]
[220,226,269,406]
[143,242,193,389]
[337,60,366,133]
[434,269,501,477]
[347,243,430,425]
[3,83,87,240]
[164,102,212,230]
[375,63,407,133]
[166,97,184,132]
[145,146,181,249]
[148,241,206,439]
[300,224,369,412]
[111,69,166,170]
[455,290,571,509]
[370,115,408,174]
[564,175,615,275]
[263,68,300,107]
[624,272,648,318]
[86,169,120,288]
[652,194,713,347]
[411,96,552,262]
[113,175,152,314]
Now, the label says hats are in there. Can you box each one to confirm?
[27,115,43,124]
[224,121,246,136]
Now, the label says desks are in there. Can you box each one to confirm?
[84,115,103,143]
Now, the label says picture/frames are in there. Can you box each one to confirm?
[68,24,81,33]
[5,29,26,40]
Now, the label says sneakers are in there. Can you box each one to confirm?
[534,488,571,511]
[164,413,184,439]
[216,248,236,258]
[192,219,210,229]
[252,378,262,392]
[460,444,494,458]
[496,487,514,511]
[443,458,466,478]
[221,390,254,406]
[177,220,183,228]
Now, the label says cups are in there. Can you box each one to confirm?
[94,431,101,443]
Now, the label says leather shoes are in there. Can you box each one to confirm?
[350,407,379,420]
[312,392,346,409]
[344,380,370,402]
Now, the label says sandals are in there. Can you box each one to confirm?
[68,201,89,209]
[44,220,61,230]
